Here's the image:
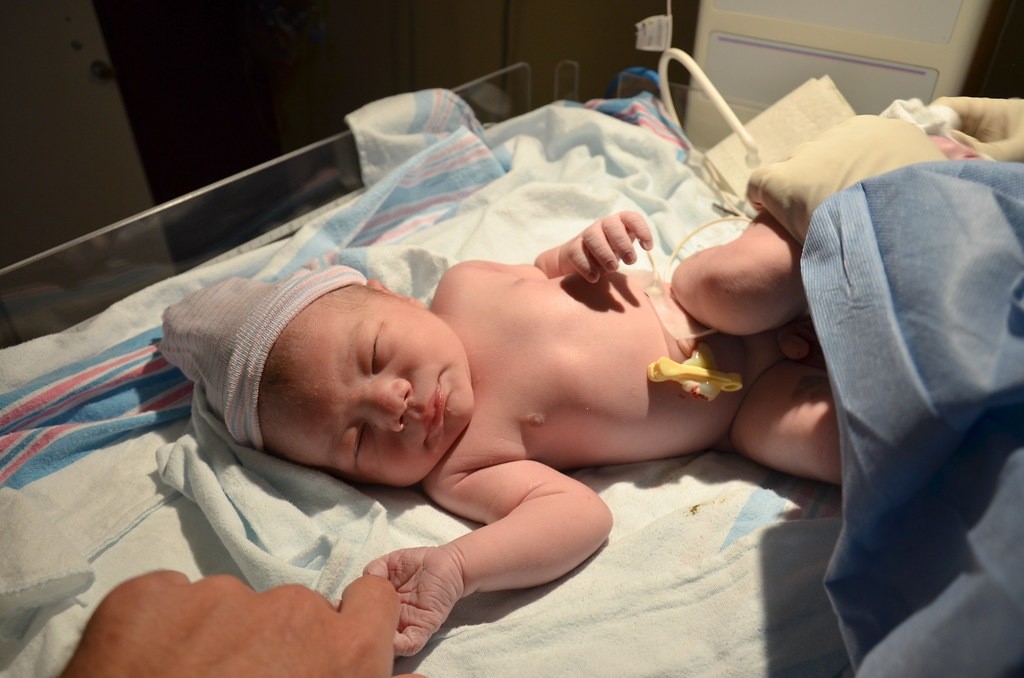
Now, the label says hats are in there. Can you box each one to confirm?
[159,266,366,450]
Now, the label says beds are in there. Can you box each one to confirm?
[0,58,888,678]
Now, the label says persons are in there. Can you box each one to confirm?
[158,134,975,656]
